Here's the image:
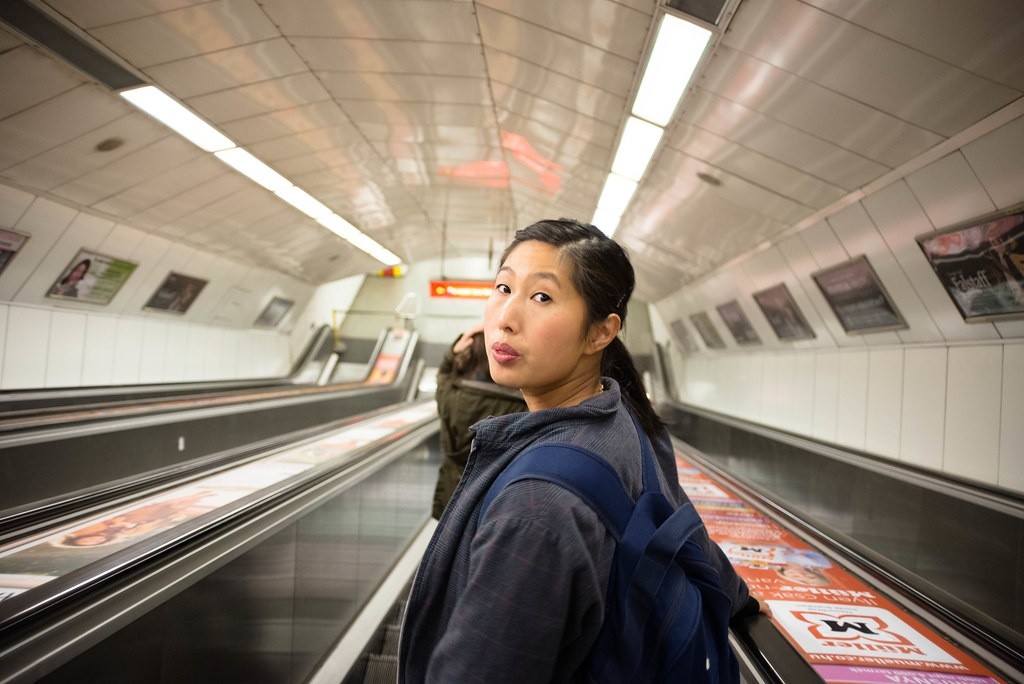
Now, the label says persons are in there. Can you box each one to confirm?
[430,327,531,523]
[52,258,91,296]
[398,213,767,682]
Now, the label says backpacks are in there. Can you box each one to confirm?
[477,391,741,684]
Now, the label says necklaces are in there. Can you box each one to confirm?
[559,384,604,406]
